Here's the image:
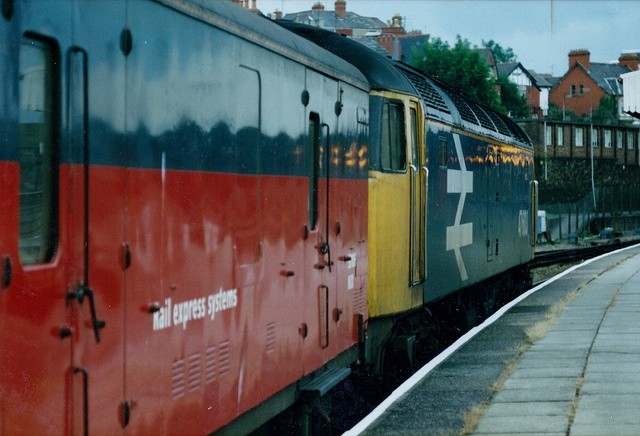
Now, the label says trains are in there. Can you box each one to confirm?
[0,1,540,435]
